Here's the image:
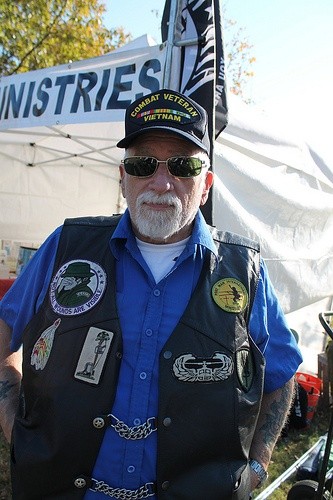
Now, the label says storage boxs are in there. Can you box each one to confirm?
[16,246,39,276]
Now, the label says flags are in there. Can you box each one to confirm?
[162,0,229,139]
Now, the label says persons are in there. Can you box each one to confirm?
[0,89,303,500]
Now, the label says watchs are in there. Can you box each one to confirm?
[249,458,270,490]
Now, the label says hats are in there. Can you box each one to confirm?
[116,89,214,156]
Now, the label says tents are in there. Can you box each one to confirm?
[0,32,333,394]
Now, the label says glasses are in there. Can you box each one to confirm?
[121,156,209,179]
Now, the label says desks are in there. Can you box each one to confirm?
[0,267,19,301]
